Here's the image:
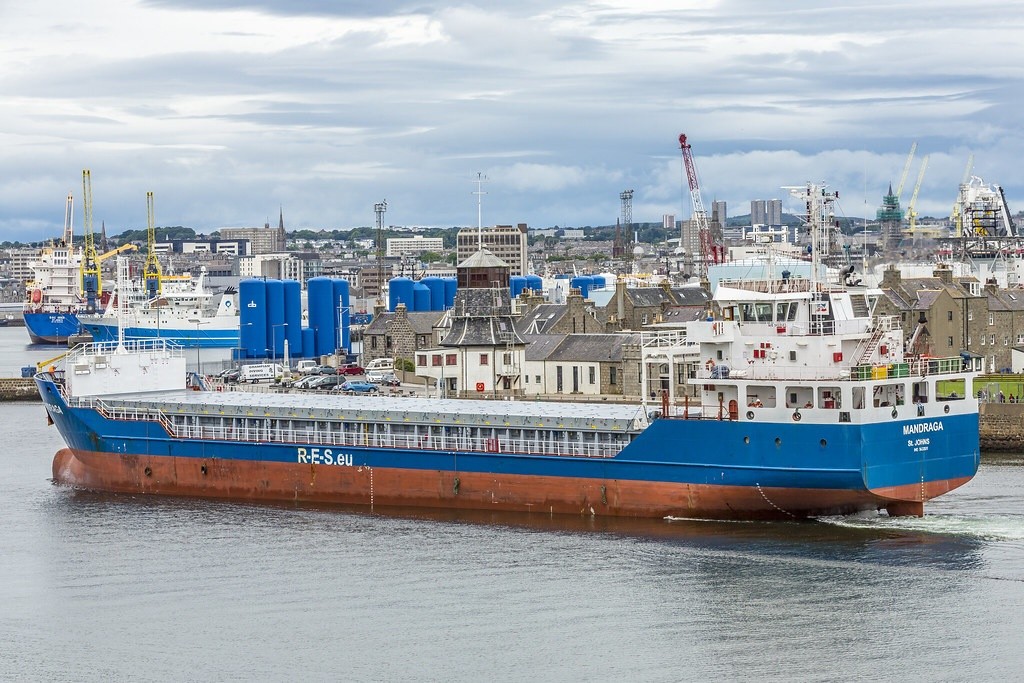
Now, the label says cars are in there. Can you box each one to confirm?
[331,380,380,393]
[290,375,322,388]
[310,365,337,376]
[365,371,384,383]
[381,373,401,386]
[309,375,348,390]
[215,367,240,383]
[335,364,364,376]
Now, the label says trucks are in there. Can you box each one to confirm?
[242,364,294,382]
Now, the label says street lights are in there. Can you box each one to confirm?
[237,322,252,385]
[273,323,288,383]
[384,320,404,383]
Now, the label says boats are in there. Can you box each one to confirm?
[24,194,121,343]
[30,253,981,519]
[74,188,243,374]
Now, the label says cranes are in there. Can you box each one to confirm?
[904,153,929,233]
[142,190,191,293]
[950,152,975,236]
[680,132,721,265]
[894,141,918,201]
[79,170,140,299]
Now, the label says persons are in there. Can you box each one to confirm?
[651,389,668,400]
[949,389,1020,403]
[756,399,763,408]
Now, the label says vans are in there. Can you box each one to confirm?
[297,360,318,376]
[365,358,394,373]
